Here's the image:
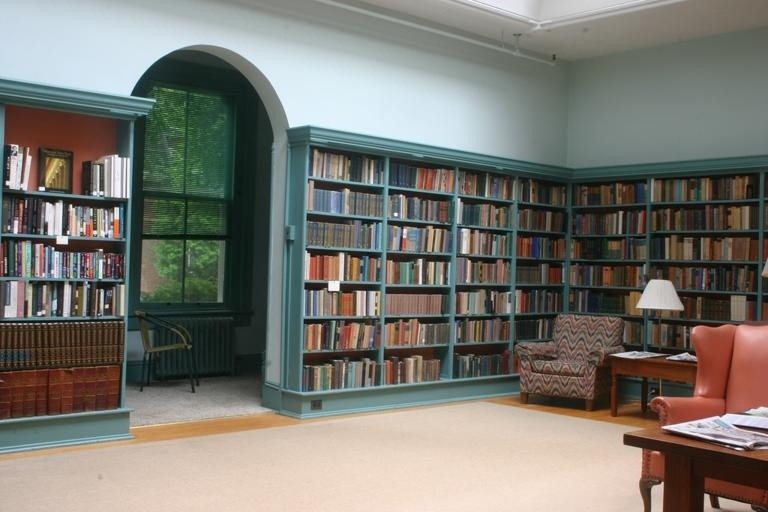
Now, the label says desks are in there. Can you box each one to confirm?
[608,353,698,418]
[622,412,768,512]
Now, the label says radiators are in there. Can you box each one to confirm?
[152,315,234,380]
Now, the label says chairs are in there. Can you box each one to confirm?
[133,309,200,394]
[638,324,768,512]
[512,312,626,412]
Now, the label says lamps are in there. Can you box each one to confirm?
[634,278,685,354]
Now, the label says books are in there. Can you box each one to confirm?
[386,162,453,385]
[0,144,130,420]
[515,180,566,341]
[570,175,758,350]
[303,151,384,394]
[455,170,514,378]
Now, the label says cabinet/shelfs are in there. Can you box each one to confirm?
[565,154,768,402]
[1,100,134,418]
[279,126,569,418]
